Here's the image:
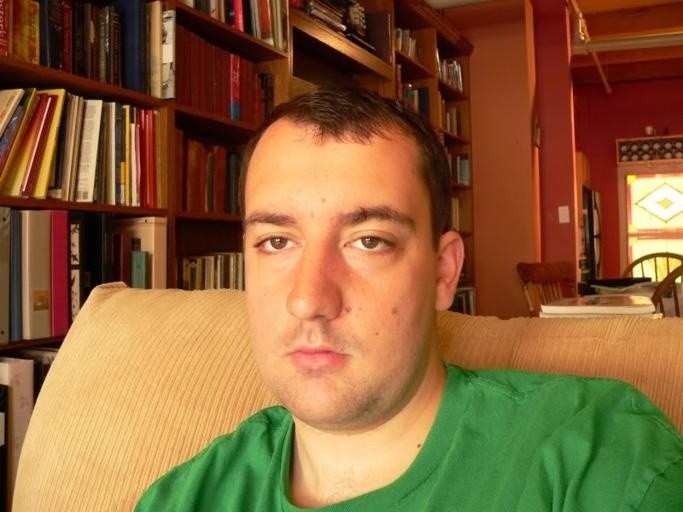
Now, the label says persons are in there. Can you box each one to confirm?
[130,89,683,512]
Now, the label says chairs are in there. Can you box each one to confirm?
[514,249,681,316]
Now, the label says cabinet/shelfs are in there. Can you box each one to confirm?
[0,0,478,356]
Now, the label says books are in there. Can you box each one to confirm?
[1,206,168,339]
[0,346,63,511]
[392,28,463,135]
[1,0,160,209]
[539,291,656,317]
[444,144,471,234]
[164,0,287,126]
[289,1,378,54]
[172,128,248,290]
